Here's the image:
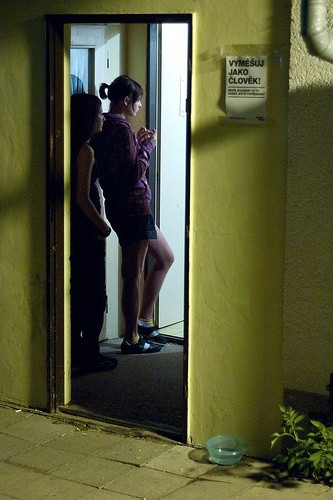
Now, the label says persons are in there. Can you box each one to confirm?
[70,86,119,372]
[93,74,175,355]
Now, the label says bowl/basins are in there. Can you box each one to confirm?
[205,435,246,465]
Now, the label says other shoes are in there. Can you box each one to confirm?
[79,352,117,373]
[137,324,167,344]
[120,335,161,354]
